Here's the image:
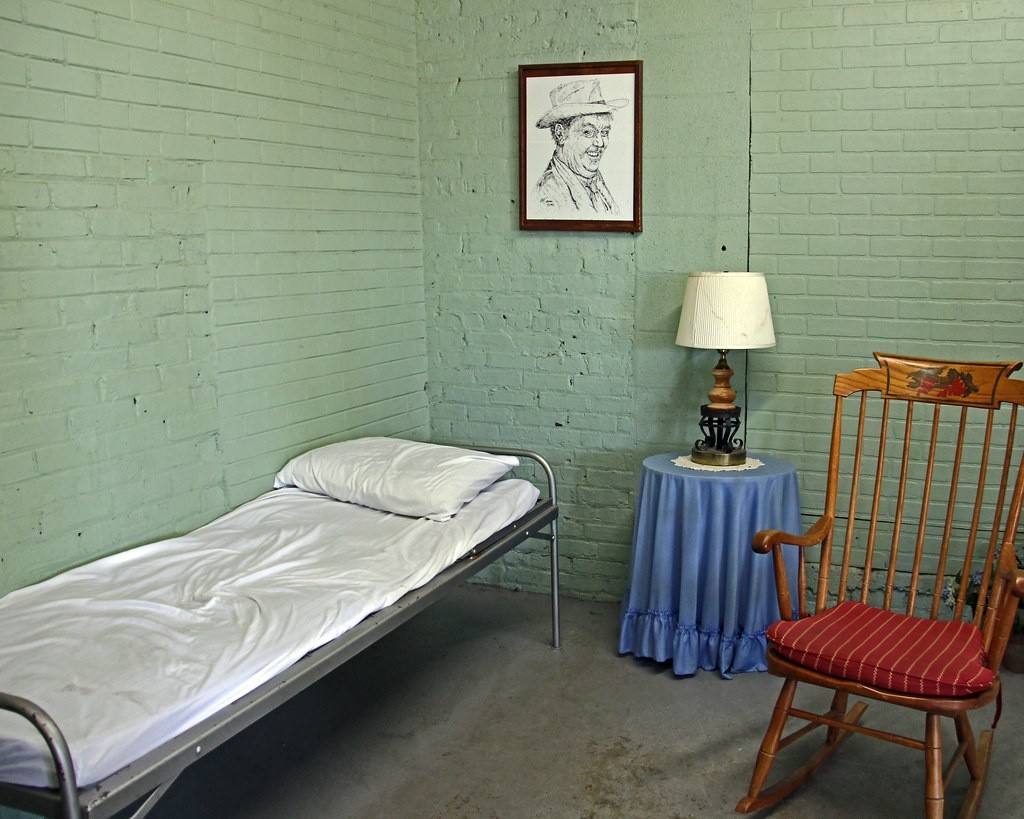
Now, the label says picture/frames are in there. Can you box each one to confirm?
[516,59,647,234]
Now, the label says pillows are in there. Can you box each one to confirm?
[271,438,518,525]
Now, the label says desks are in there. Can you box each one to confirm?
[618,449,807,677]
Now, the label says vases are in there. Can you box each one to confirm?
[982,626,1024,675]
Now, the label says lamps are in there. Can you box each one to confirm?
[668,272,780,468]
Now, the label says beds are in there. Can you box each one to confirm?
[0,434,568,817]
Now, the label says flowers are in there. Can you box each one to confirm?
[943,544,1024,634]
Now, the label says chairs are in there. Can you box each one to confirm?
[734,349,1024,816]
